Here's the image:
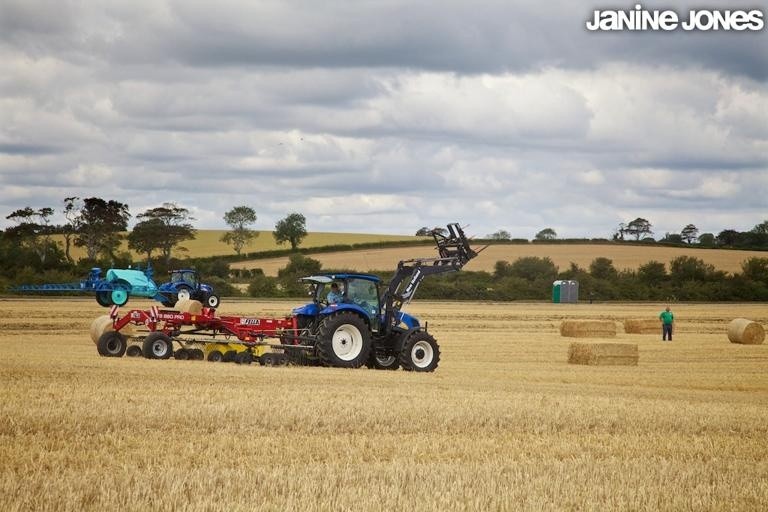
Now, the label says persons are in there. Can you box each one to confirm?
[326,282,344,305]
[658,307,674,341]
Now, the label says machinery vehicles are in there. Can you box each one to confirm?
[98,223,489,373]
[95,268,220,309]
